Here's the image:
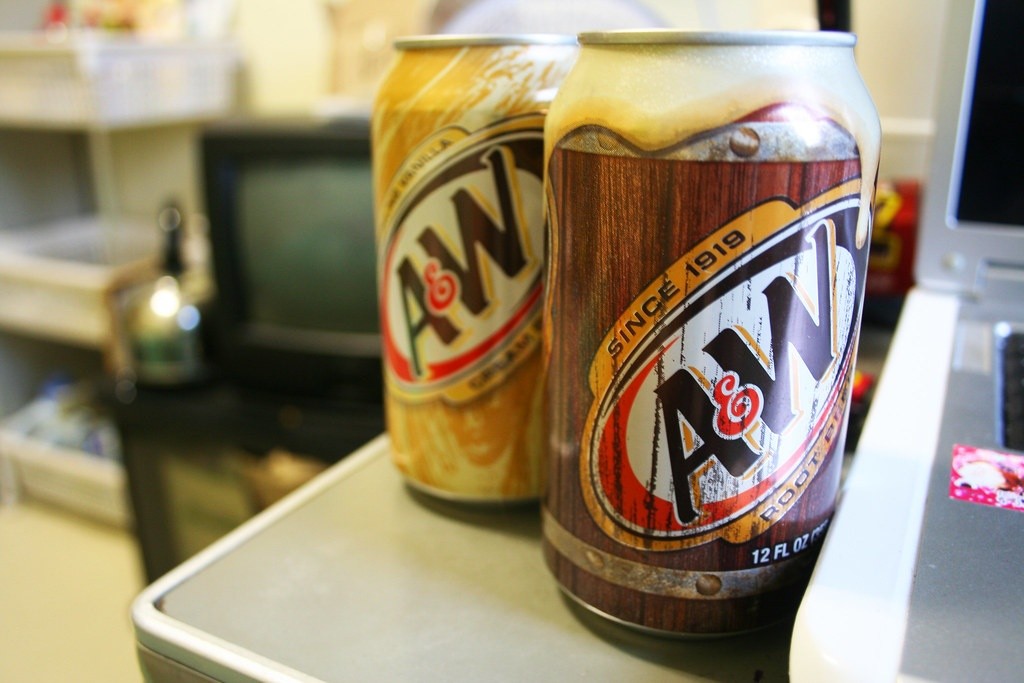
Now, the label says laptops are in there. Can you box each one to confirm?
[786,0,1024,683]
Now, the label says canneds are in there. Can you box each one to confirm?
[537,30,882,640]
[367,32,583,507]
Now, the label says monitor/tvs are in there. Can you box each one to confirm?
[198,121,386,411]
